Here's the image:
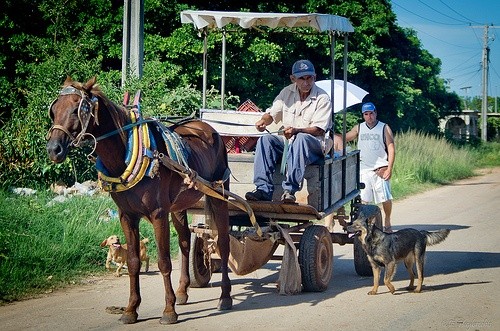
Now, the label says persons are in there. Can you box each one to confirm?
[245,61,334,203]
[341,104,394,235]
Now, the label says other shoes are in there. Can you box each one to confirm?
[281,190,296,203]
[245,189,273,201]
[385,226,392,232]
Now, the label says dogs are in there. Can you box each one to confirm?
[100,235,150,277]
[343,211,450,296]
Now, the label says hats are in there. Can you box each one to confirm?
[292,59,316,79]
[361,102,376,113]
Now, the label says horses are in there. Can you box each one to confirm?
[47,75,233,324]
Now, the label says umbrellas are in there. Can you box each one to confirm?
[315,79,369,113]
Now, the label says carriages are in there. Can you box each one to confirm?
[45,9,383,325]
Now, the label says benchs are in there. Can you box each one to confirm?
[199,109,320,211]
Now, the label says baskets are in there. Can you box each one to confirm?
[222,100,262,154]
